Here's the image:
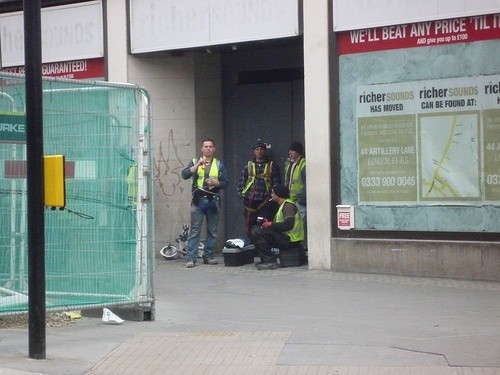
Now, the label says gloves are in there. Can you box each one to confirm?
[262,222,272,230]
[257,216,268,224]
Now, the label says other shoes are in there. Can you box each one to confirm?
[203,257,217,265]
[255,261,278,269]
[185,260,195,268]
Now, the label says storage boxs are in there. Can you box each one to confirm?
[221,244,256,266]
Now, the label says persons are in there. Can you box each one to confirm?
[250,182,305,270]
[182,138,229,268]
[238,141,281,244]
[283,142,306,250]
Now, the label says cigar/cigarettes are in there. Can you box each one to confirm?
[269,198,273,201]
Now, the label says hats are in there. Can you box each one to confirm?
[290,142,303,153]
[253,139,266,151]
[273,183,290,199]
